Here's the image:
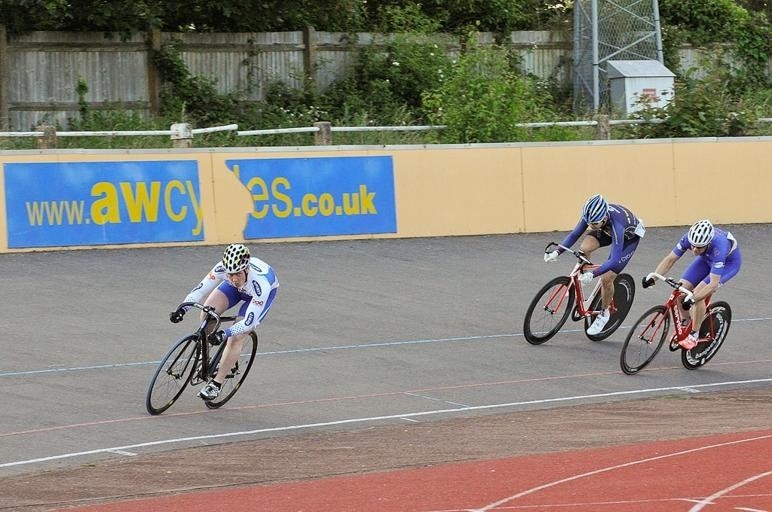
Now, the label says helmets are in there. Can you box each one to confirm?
[688,220,714,248]
[583,195,608,224]
[222,244,250,274]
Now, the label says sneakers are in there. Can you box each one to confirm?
[679,334,699,350]
[201,382,222,400]
[586,314,610,335]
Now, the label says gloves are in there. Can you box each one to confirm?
[681,297,691,311]
[544,251,558,263]
[170,309,185,323]
[642,277,655,288]
[579,272,593,284]
[209,330,226,345]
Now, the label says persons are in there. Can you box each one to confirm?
[543,193,647,337]
[641,219,742,351]
[170,243,280,400]
